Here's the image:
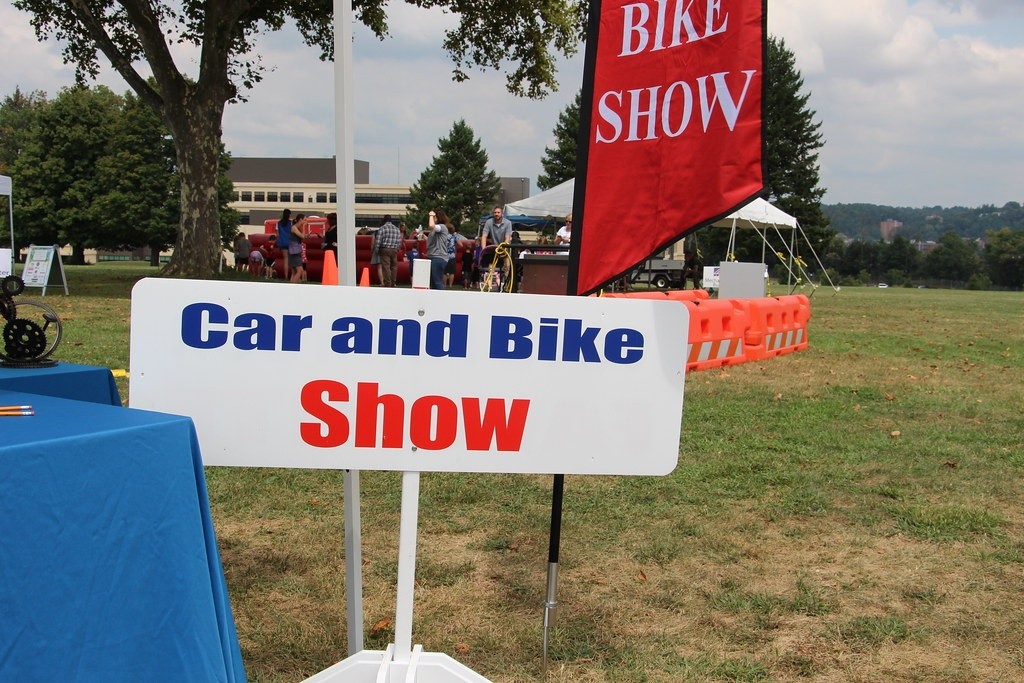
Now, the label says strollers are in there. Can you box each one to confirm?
[473,245,506,292]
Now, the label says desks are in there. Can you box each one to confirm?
[1,364,248,683]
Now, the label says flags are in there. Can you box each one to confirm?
[576,1,767,294]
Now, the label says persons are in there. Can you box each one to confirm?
[427,210,449,290]
[408,243,418,284]
[519,249,531,259]
[233,233,251,271]
[316,213,338,266]
[259,235,276,279]
[462,237,482,289]
[276,209,294,280]
[536,239,553,254]
[414,228,427,239]
[248,251,264,276]
[374,215,401,287]
[554,214,573,255]
[481,207,512,280]
[444,224,463,288]
[509,232,522,288]
[290,213,312,283]
[400,224,407,257]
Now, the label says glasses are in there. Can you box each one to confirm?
[565,220,572,223]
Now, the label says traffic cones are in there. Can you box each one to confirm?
[360,267,370,287]
[321,250,339,286]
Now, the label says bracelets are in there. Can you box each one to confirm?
[568,238,570,243]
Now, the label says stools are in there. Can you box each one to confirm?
[685,277,703,290]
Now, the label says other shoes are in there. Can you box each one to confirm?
[379,284,385,287]
[385,284,392,287]
[392,283,397,287]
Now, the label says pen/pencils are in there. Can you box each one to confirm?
[0,405,35,416]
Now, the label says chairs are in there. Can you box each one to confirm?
[478,248,506,292]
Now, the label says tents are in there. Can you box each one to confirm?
[502,177,841,298]
